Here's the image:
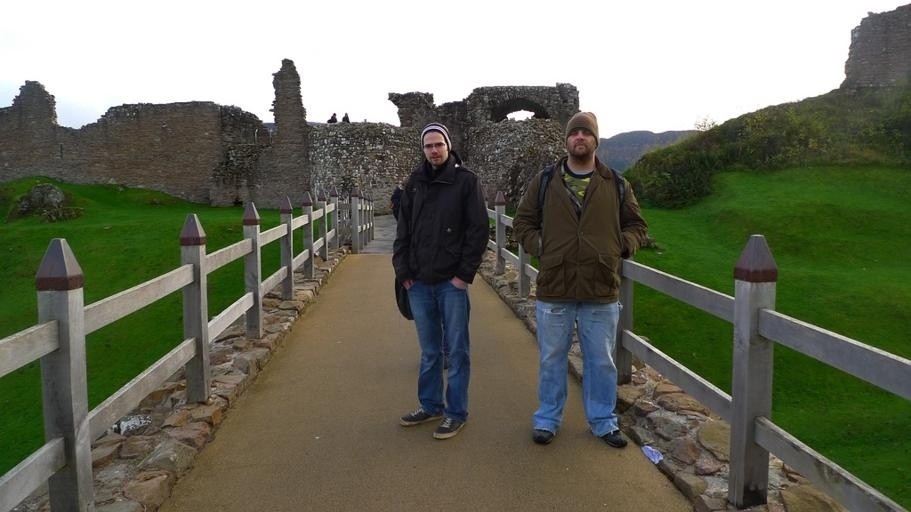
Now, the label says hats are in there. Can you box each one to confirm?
[420,122,452,153]
[564,112,599,148]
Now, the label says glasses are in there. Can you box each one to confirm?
[423,142,446,148]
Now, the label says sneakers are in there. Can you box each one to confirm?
[533,429,554,445]
[602,429,628,448]
[433,416,465,439]
[400,406,443,426]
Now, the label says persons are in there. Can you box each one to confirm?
[512,112,647,448]
[328,113,337,123]
[390,124,490,438]
[343,113,350,121]
